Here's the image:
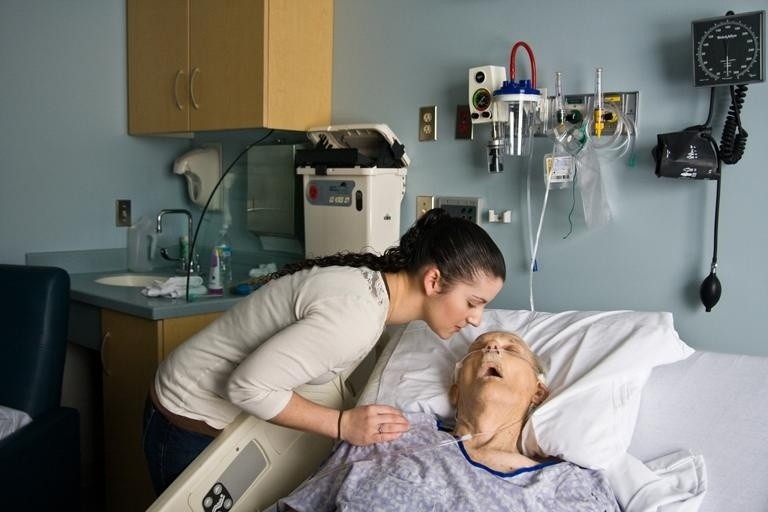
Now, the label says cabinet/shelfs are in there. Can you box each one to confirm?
[61,265,245,512]
[126,0,334,140]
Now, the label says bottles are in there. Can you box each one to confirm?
[208,224,233,295]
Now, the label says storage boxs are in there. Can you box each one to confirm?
[296,124,411,259]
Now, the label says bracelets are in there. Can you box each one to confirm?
[337,408,343,440]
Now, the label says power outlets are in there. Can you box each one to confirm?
[419,105,437,142]
[551,95,588,137]
[517,96,554,136]
[115,200,132,227]
[455,104,474,141]
[439,196,483,226]
[415,196,435,220]
[491,104,529,138]
[588,90,638,138]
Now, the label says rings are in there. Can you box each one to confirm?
[378,423,385,434]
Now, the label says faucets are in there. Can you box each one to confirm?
[155,209,200,275]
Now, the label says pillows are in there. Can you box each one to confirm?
[353,309,695,471]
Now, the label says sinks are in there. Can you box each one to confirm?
[96,275,170,288]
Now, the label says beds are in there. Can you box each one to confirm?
[145,350,768,511]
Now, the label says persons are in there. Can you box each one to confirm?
[278,331,621,512]
[141,208,506,499]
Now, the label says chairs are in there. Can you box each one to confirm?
[1,264,81,482]
